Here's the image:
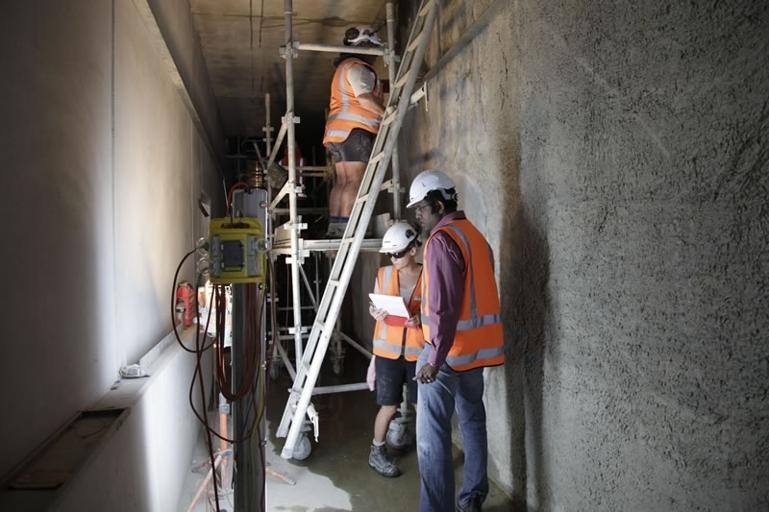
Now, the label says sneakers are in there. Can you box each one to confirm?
[368,440,400,476]
[324,222,373,236]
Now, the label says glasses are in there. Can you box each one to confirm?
[383,253,404,258]
[412,203,431,213]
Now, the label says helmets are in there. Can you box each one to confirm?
[378,222,422,253]
[406,169,456,209]
[346,24,381,46]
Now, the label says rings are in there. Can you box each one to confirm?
[426,379,431,384]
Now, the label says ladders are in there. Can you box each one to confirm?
[272,0,436,458]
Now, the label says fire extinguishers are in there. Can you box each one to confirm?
[177,283,195,329]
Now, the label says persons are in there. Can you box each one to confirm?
[367,219,423,478]
[404,166,507,512]
[322,21,427,240]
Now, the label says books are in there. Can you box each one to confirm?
[368,292,413,319]
